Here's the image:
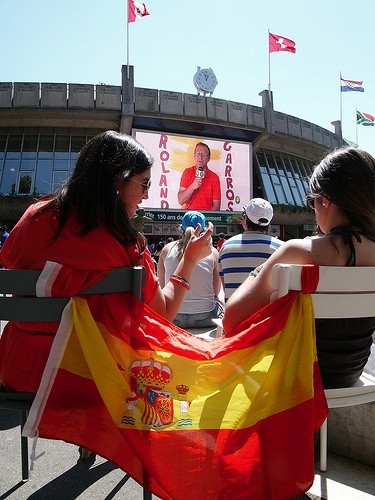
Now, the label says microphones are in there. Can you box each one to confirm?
[195,167,204,190]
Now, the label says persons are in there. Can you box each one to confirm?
[0,130,375,460]
[177,142,221,211]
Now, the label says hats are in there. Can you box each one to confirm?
[182,211,205,232]
[245,198,273,226]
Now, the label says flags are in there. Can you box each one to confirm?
[357,110,375,127]
[341,78,365,92]
[269,33,296,54]
[21,260,329,500]
[127,0,150,23]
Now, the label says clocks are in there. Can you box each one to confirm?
[193,66,218,97]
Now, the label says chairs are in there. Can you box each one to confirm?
[0,264,153,500]
[211,262,375,500]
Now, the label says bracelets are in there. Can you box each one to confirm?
[249,265,263,278]
[168,274,191,290]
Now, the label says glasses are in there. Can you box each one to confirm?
[306,195,322,210]
[194,152,209,157]
[127,178,151,194]
